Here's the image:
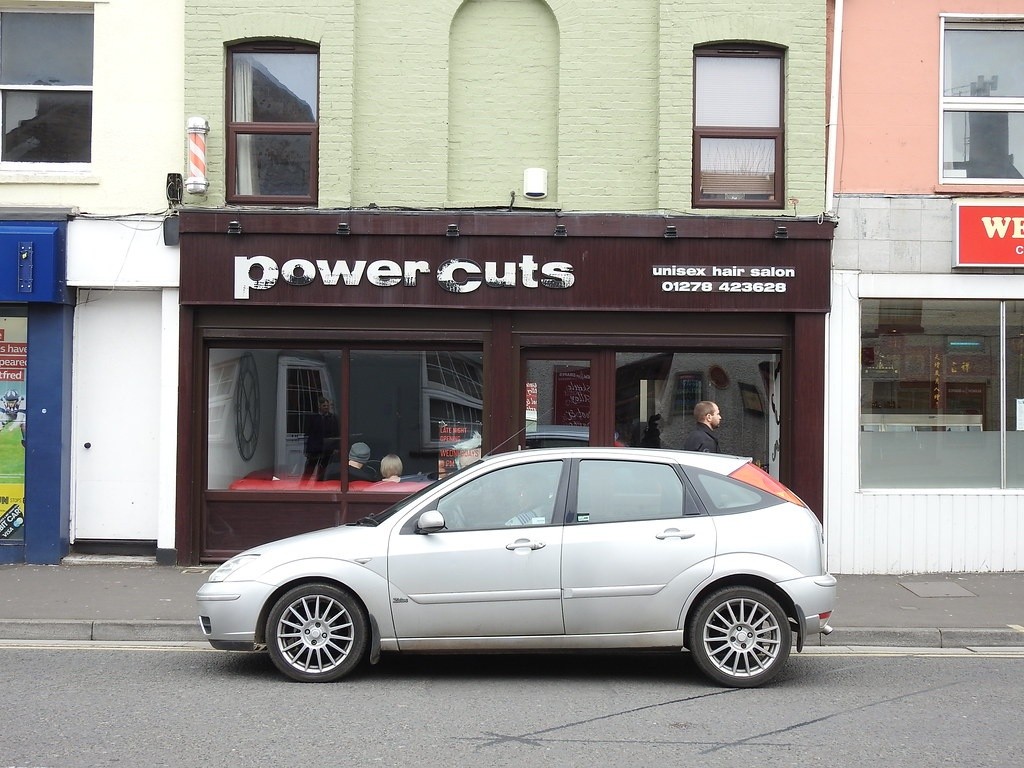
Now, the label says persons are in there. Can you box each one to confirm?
[325,442,403,484]
[683,400,722,454]
[299,398,339,489]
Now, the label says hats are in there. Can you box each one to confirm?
[348,442,370,464]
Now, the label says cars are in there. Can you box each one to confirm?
[193,447,839,688]
[363,423,633,492]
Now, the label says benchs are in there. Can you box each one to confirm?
[229,478,429,493]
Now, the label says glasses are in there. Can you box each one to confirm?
[322,404,329,408]
